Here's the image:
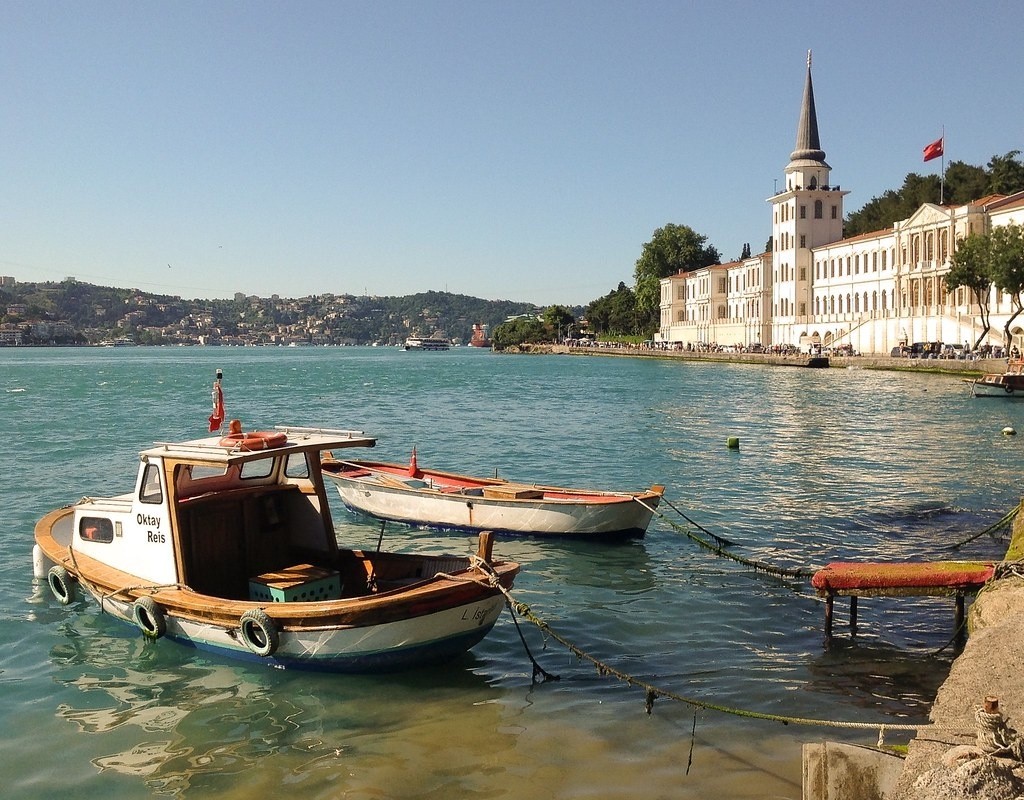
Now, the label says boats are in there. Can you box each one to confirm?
[318,451,666,541]
[962,351,1024,397]
[114,339,137,346]
[32,369,520,674]
[402,337,451,351]
[471,324,496,347]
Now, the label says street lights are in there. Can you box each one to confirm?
[802,288,808,332]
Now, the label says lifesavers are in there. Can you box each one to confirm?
[217,427,291,453]
[238,607,281,659]
[45,562,78,607]
[1005,383,1015,394]
[130,592,170,642]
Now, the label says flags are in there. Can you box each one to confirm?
[208,386,225,433]
[922,137,943,162]
[409,451,417,477]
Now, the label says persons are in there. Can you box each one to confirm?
[552,338,855,355]
[900,340,1019,359]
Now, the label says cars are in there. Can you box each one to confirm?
[663,344,684,352]
[715,345,728,353]
[891,346,912,357]
[692,343,710,352]
[823,347,837,355]
[974,345,1002,357]
[764,345,783,353]
[599,342,606,348]
[907,342,944,359]
[833,348,855,356]
[943,344,964,355]
[750,343,763,353]
[727,345,748,353]
